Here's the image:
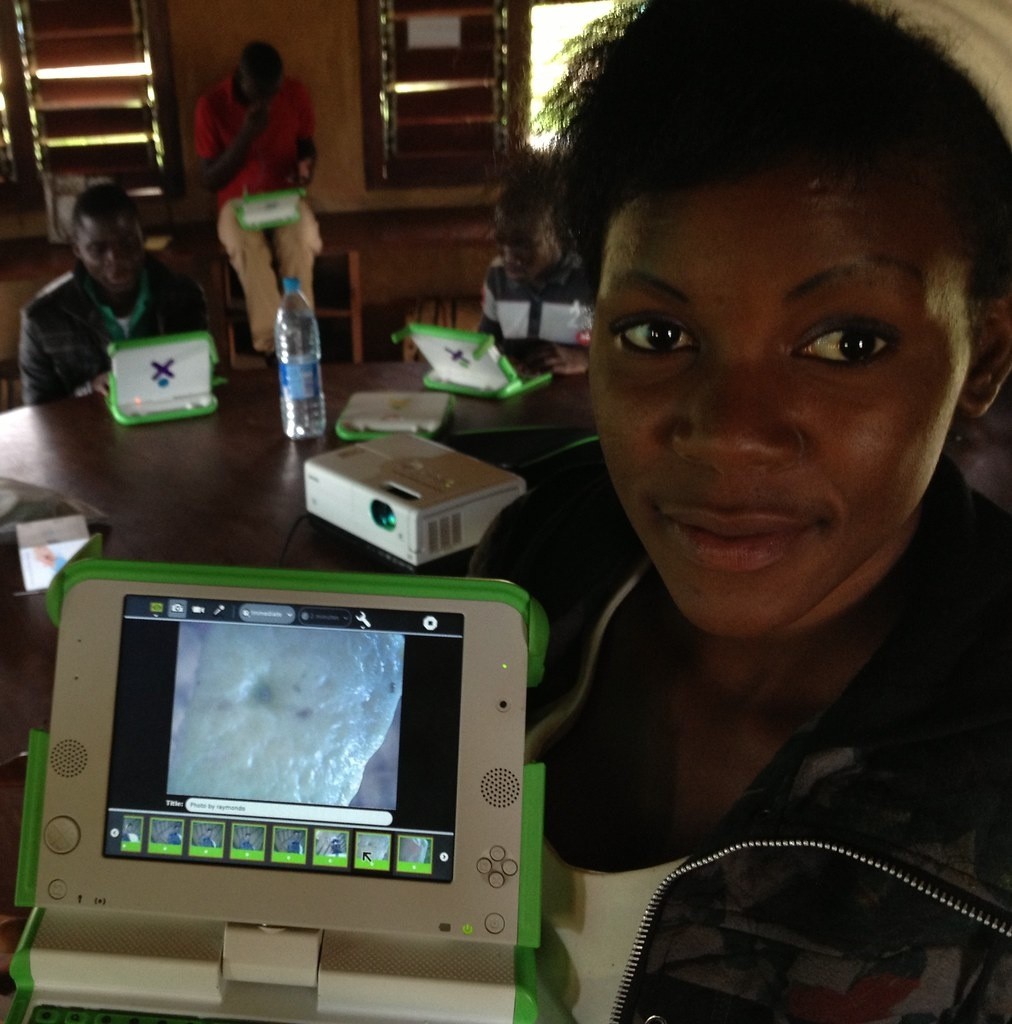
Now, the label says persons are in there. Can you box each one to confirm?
[474,0,1012,1024]
[18,183,210,404]
[478,182,594,376]
[194,40,319,365]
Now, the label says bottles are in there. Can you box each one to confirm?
[274,277,327,440]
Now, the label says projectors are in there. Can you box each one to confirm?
[304,430,530,565]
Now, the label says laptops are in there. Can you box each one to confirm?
[231,187,307,232]
[102,329,228,427]
[335,322,551,443]
[0,558,550,1024]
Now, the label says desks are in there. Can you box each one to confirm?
[0,360,1012,1024]
[0,185,501,361]
